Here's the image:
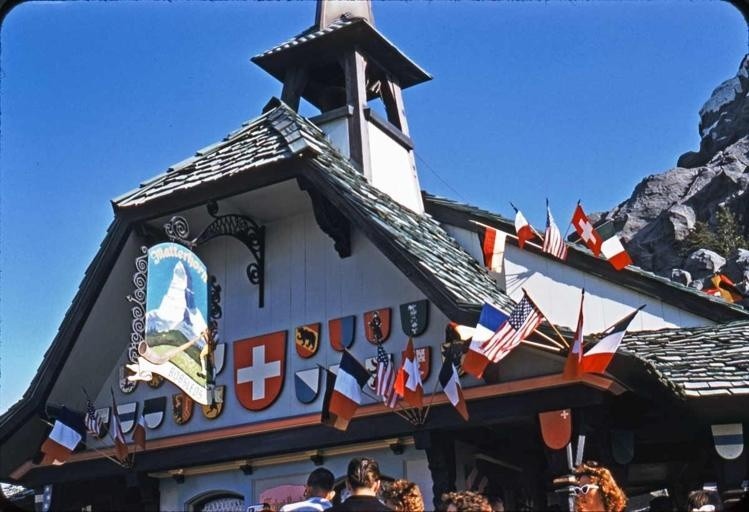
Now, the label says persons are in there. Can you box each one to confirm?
[440,488,492,511]
[387,479,425,512]
[649,495,674,511]
[280,467,336,512]
[686,490,709,512]
[195,321,218,386]
[574,463,629,512]
[323,456,395,511]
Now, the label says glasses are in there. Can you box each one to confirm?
[571,483,599,494]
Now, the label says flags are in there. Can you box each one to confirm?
[710,273,745,304]
[320,370,351,432]
[460,304,510,381]
[564,287,585,380]
[374,341,399,410]
[600,233,633,272]
[438,341,470,421]
[477,293,544,364]
[543,206,567,261]
[328,352,371,423]
[393,333,425,412]
[31,390,150,467]
[483,227,508,273]
[572,206,603,257]
[584,304,645,375]
[510,203,535,250]
